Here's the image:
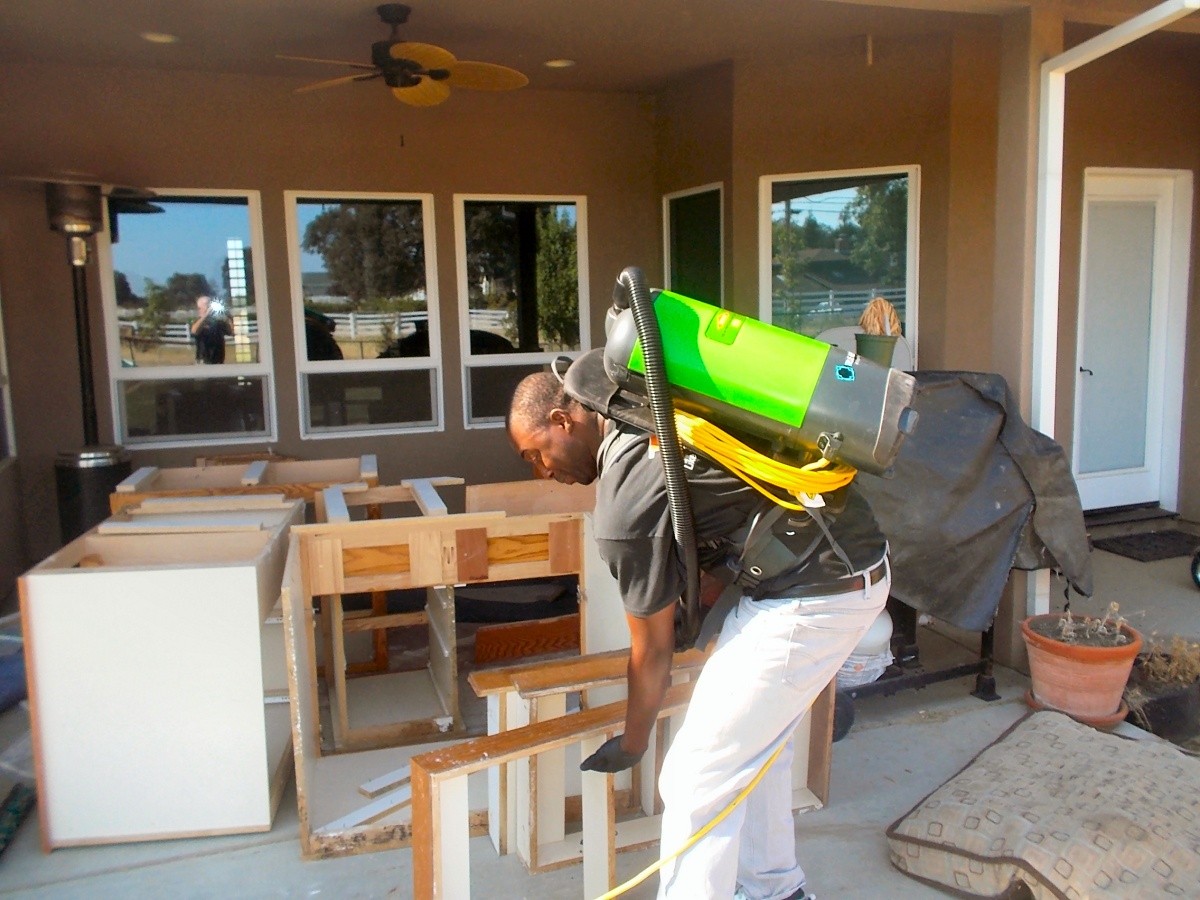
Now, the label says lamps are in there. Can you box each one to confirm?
[46,183,130,547]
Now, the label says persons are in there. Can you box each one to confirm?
[190,296,234,364]
[506,373,892,900]
[305,309,343,361]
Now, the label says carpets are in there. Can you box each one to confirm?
[1092,529,1200,563]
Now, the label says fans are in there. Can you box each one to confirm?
[276,5,528,149]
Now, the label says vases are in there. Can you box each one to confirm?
[854,333,898,369]
[1022,613,1147,718]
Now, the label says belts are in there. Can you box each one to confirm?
[763,554,892,600]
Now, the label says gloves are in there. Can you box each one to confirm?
[580,735,650,773]
